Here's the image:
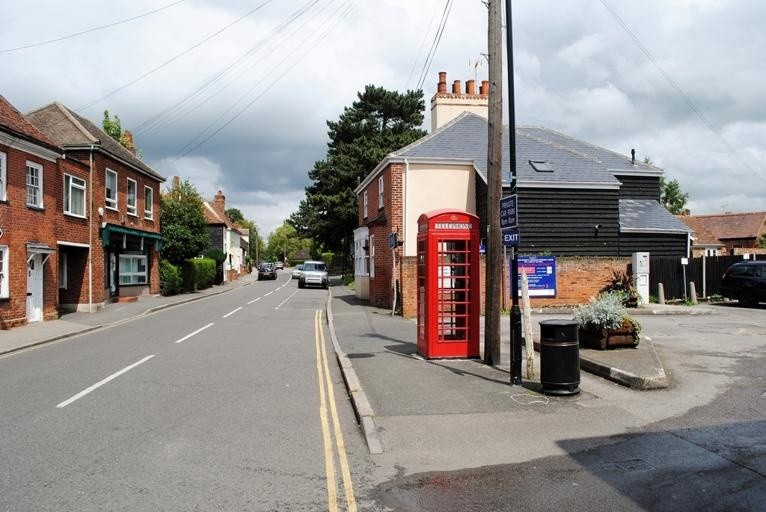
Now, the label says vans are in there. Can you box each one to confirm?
[719,261,766,307]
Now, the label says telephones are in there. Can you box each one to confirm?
[451,254,464,276]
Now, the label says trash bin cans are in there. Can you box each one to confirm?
[538,319,581,395]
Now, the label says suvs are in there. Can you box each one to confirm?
[297,260,329,289]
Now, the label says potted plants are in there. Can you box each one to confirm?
[570,290,640,350]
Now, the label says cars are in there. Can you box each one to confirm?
[291,265,303,279]
[257,260,284,281]
